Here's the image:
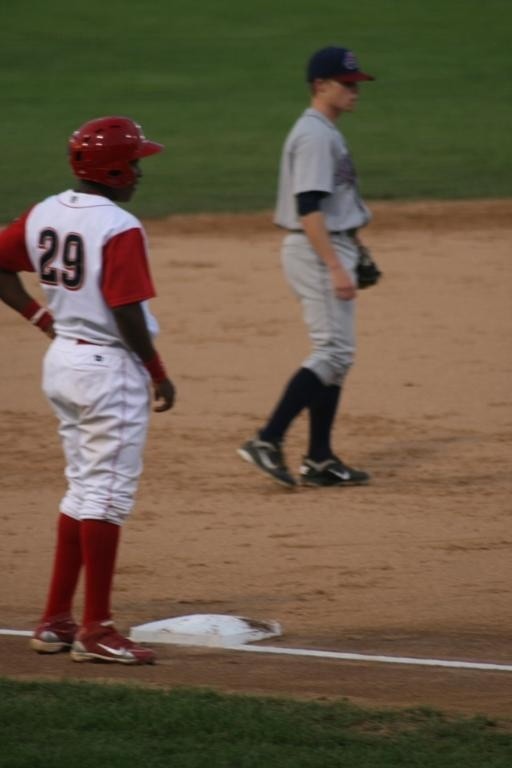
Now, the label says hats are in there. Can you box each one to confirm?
[304,43,377,85]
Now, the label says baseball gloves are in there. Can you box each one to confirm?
[357,246,379,287]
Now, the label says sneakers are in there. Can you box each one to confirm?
[299,457,370,488]
[234,432,298,488]
[68,623,159,667]
[28,618,82,655]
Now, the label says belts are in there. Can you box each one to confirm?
[78,336,131,349]
[287,225,358,238]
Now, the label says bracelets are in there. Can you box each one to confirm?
[143,352,166,384]
[21,299,54,331]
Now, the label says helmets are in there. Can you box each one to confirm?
[67,114,167,191]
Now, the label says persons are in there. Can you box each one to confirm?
[1,118,177,667]
[238,45,378,488]
